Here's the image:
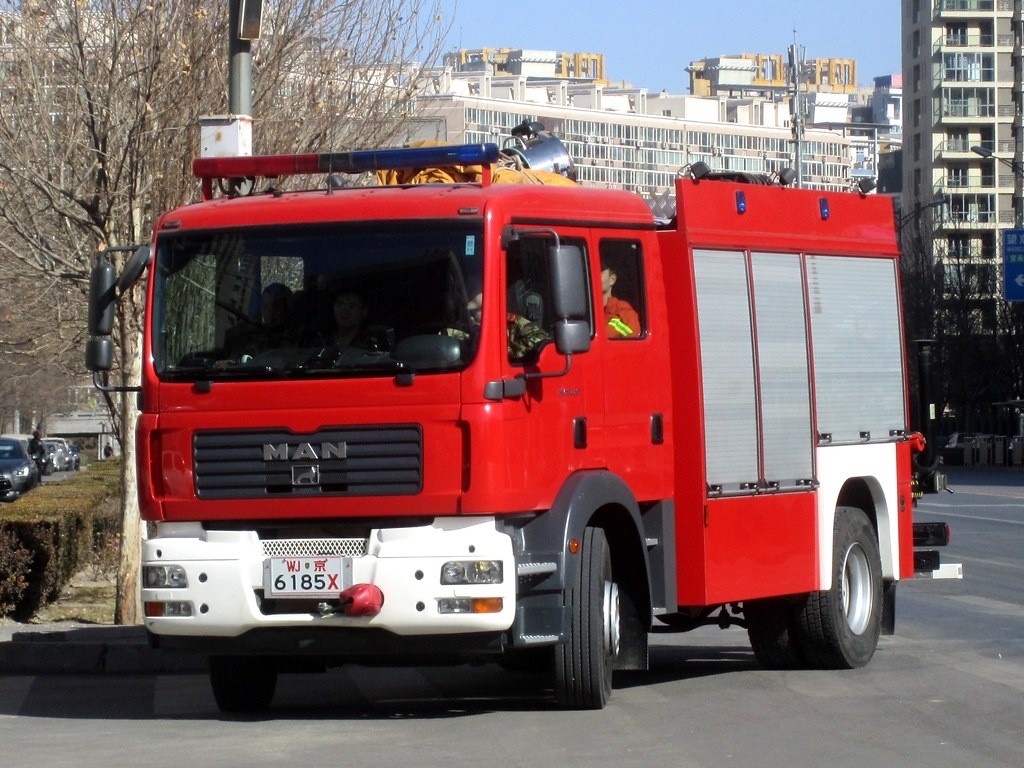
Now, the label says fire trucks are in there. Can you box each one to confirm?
[85,116,949,721]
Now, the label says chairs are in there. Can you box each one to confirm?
[508,287,544,329]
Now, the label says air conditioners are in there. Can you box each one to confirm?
[487,0,1010,223]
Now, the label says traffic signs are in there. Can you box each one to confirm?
[1001,228,1024,303]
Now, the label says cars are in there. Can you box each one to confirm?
[0,436,81,503]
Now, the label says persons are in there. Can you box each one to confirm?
[230,284,315,359]
[104,442,113,458]
[27,431,45,484]
[321,284,390,351]
[600,260,641,338]
[438,267,552,360]
